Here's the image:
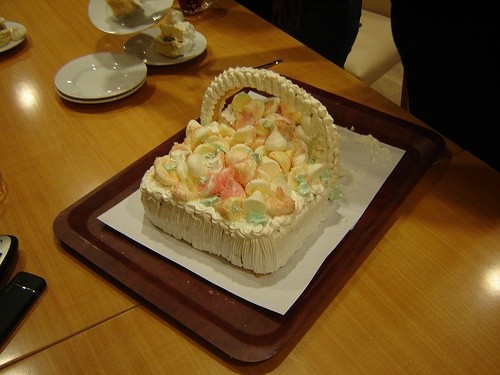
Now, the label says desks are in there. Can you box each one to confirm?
[0,0,500,375]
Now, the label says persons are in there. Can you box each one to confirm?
[233,0,500,174]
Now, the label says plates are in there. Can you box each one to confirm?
[123,28,207,65]
[88,0,173,35]
[0,22,26,53]
[54,52,147,104]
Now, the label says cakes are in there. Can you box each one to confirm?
[140,66,343,275]
[105,0,140,21]
[0,17,26,48]
[154,10,196,58]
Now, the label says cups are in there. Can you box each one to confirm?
[178,0,209,21]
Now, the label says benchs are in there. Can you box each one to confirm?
[343,0,403,106]
